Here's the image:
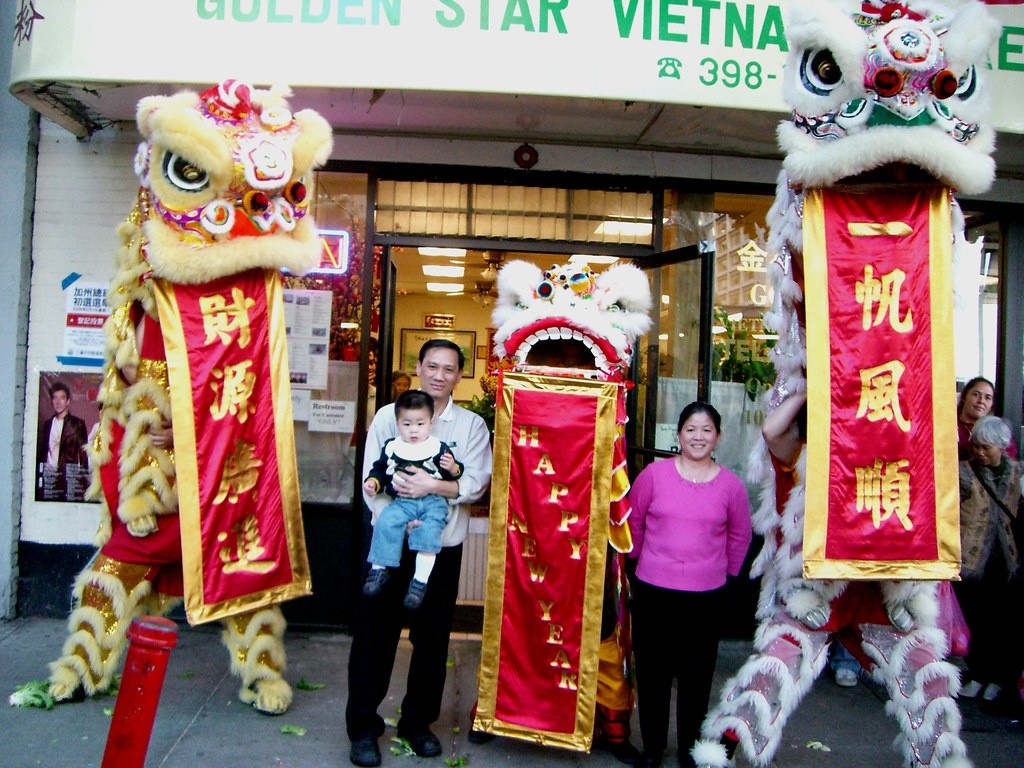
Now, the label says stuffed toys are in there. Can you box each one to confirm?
[10,80,334,713]
[468,260,654,768]
[688,0,996,768]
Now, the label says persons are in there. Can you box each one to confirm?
[344,337,492,768]
[36,382,104,502]
[625,400,753,768]
[826,376,1024,732]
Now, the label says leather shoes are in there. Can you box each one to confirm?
[349,735,382,767]
[397,729,442,757]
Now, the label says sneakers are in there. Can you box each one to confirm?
[402,579,427,608]
[362,568,390,596]
[836,668,857,686]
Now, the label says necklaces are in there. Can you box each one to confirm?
[680,457,711,484]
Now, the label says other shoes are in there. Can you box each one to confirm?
[634,751,664,768]
[600,736,641,765]
[959,679,982,698]
[677,751,698,768]
[469,727,495,744]
[982,683,1002,700]
